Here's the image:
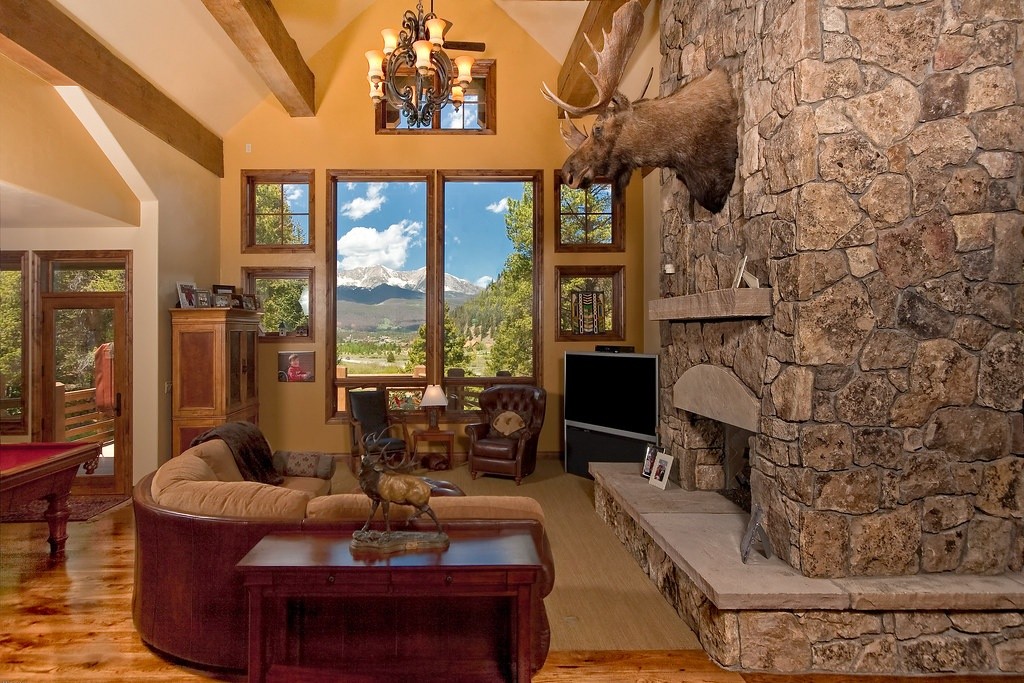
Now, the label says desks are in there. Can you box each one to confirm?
[235,528,542,683]
[412,431,455,470]
[0,440,103,552]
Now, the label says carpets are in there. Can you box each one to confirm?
[0,494,131,522]
[401,464,702,652]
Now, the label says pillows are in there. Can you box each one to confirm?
[271,450,337,480]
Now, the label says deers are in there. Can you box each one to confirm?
[537,2,740,213]
[348,423,467,535]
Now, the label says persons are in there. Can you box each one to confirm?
[184,289,194,306]
[288,354,311,382]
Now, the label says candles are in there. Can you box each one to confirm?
[664,263,675,274]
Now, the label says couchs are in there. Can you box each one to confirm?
[132,420,552,683]
[465,385,547,485]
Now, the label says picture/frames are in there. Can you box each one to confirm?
[641,443,666,479]
[731,256,760,288]
[650,452,674,490]
[741,503,770,563]
[176,280,260,310]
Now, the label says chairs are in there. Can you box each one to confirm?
[345,383,414,480]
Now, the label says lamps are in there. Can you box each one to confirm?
[365,0,474,127]
[420,385,449,432]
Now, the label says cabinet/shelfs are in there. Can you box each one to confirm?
[168,307,265,458]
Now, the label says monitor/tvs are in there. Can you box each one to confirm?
[563,351,660,444]
